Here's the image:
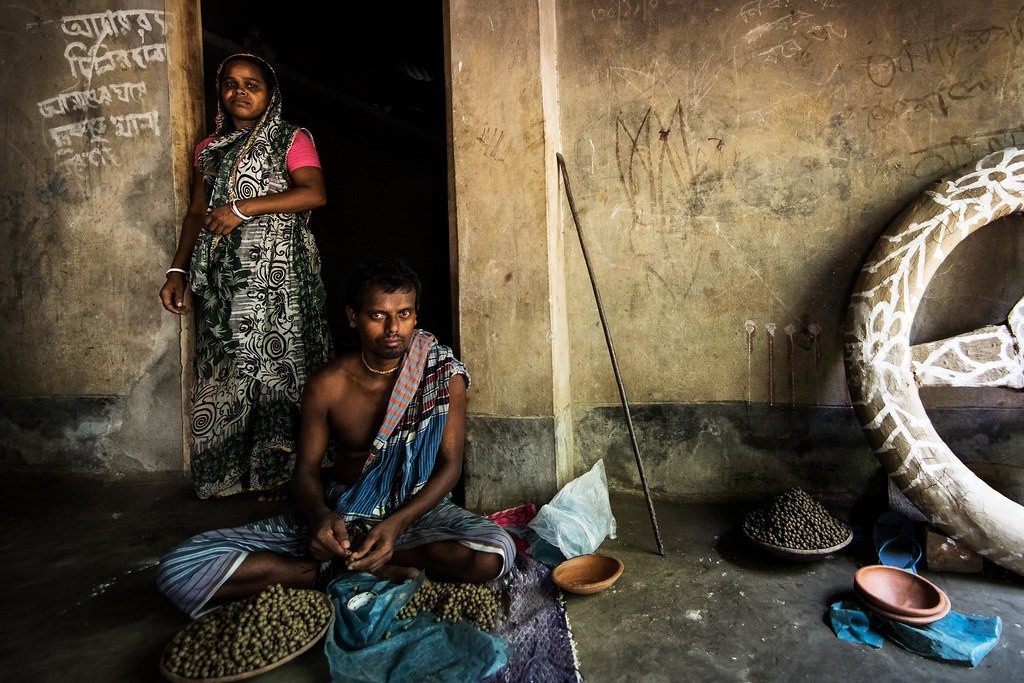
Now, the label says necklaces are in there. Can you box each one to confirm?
[361,349,402,374]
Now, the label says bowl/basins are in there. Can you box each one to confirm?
[551,553,625,594]
[160,591,336,683]
[856,564,945,616]
[742,519,854,564]
[857,591,952,626]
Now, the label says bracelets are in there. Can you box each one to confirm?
[227,200,239,217]
[164,268,189,282]
[231,197,253,220]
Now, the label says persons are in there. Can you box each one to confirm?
[159,55,330,505]
[156,265,516,610]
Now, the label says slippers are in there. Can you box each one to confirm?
[873,511,922,575]
